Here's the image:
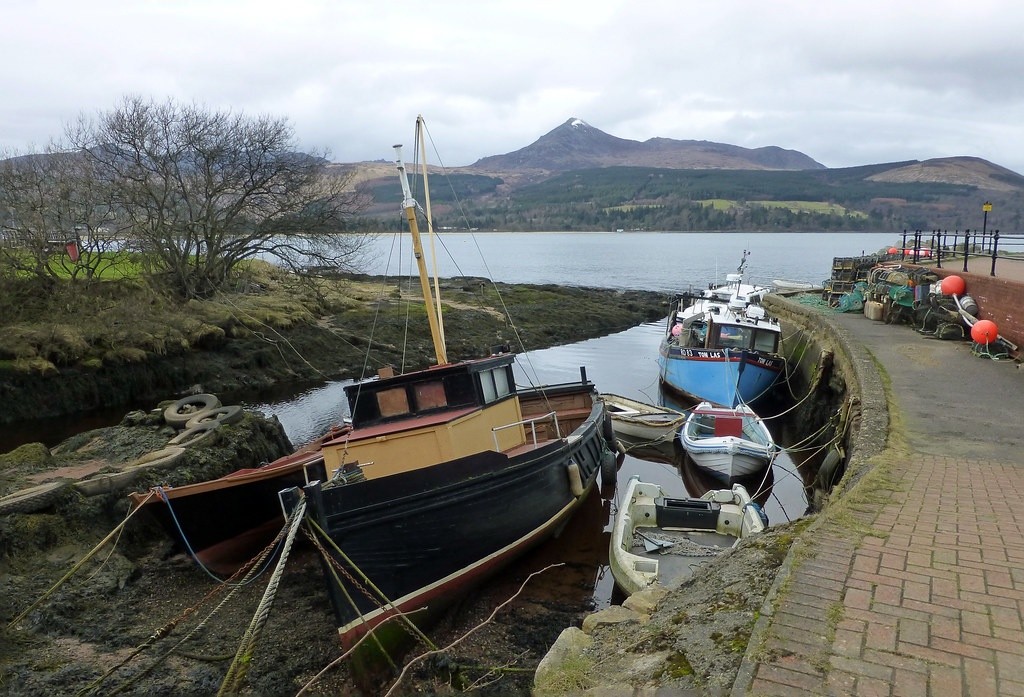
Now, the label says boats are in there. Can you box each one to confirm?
[773,280,819,291]
[608,474,767,597]
[680,400,776,487]
[127,120,618,594]
[658,251,787,409]
[599,393,684,444]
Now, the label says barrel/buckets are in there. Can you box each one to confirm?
[960,295,978,316]
[863,300,884,321]
